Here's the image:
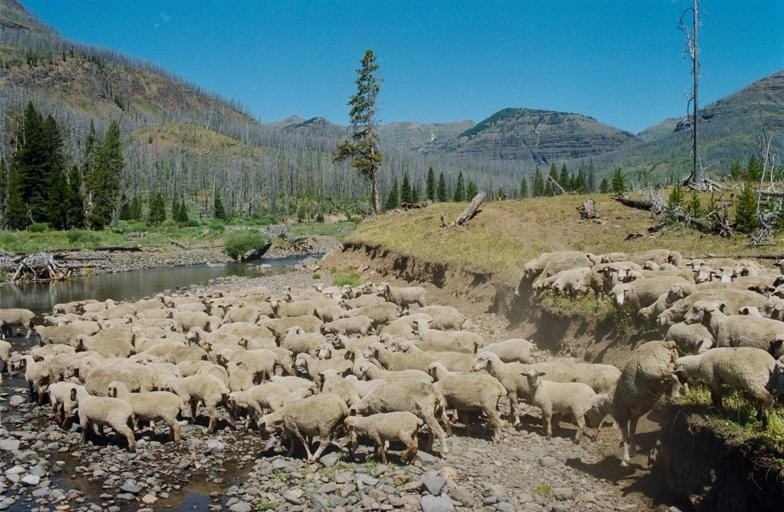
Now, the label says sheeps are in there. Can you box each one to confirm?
[0,249,779,473]
